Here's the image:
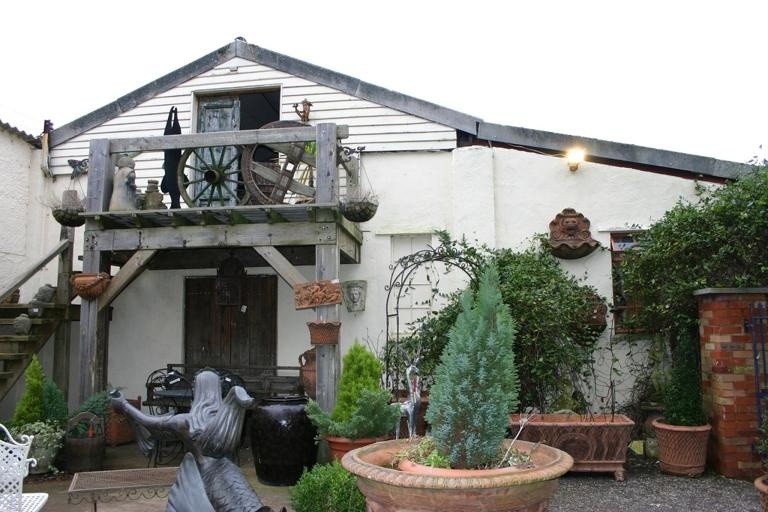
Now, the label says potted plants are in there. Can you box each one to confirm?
[3,419,66,474]
[306,256,712,511]
[62,381,128,475]
[753,392,768,512]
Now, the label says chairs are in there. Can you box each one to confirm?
[0,458,37,482]
[0,424,49,512]
[146,369,246,469]
[104,395,141,448]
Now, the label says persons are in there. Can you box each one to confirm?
[111,370,264,512]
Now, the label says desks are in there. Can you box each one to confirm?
[68,467,179,512]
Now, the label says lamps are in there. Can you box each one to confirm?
[292,98,313,122]
[566,144,586,172]
